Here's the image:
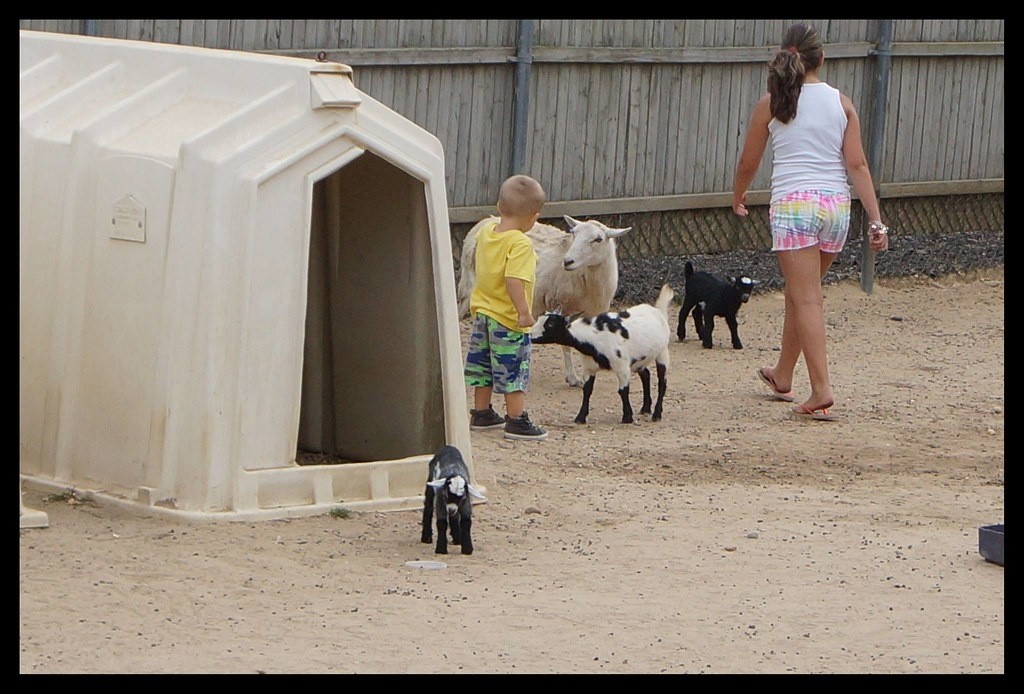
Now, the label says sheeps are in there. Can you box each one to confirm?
[676,261,761,350]
[454,213,633,388]
[420,446,485,555]
[530,285,675,425]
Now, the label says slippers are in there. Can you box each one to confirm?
[757,367,794,401]
[788,404,839,419]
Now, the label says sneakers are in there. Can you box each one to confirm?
[504,411,548,440]
[470,403,506,431]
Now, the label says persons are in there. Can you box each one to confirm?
[464,175,551,439]
[732,22,892,416]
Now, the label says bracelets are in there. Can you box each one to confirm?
[867,220,889,235]
